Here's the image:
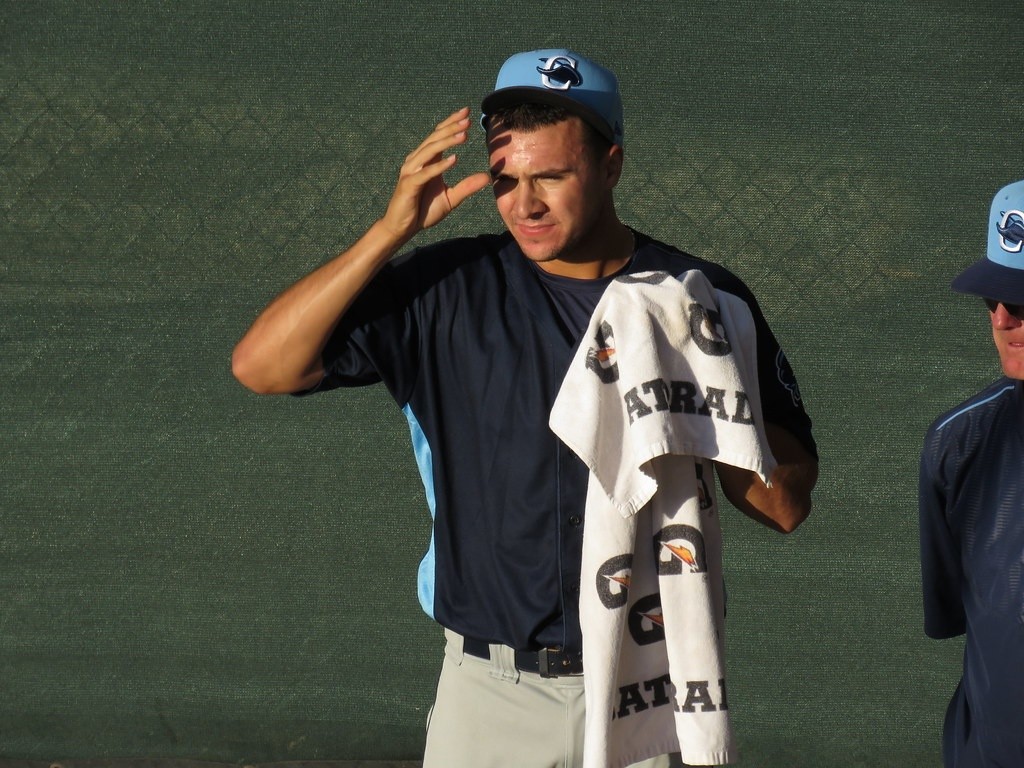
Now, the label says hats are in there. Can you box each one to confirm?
[479,49,624,148]
[950,180,1024,305]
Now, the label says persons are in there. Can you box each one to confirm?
[918,180,1024,768]
[229,48,816,768]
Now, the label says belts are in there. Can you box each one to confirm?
[463,637,584,675]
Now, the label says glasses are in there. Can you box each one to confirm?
[981,296,1024,317]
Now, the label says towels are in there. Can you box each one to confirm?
[547,268,779,768]
